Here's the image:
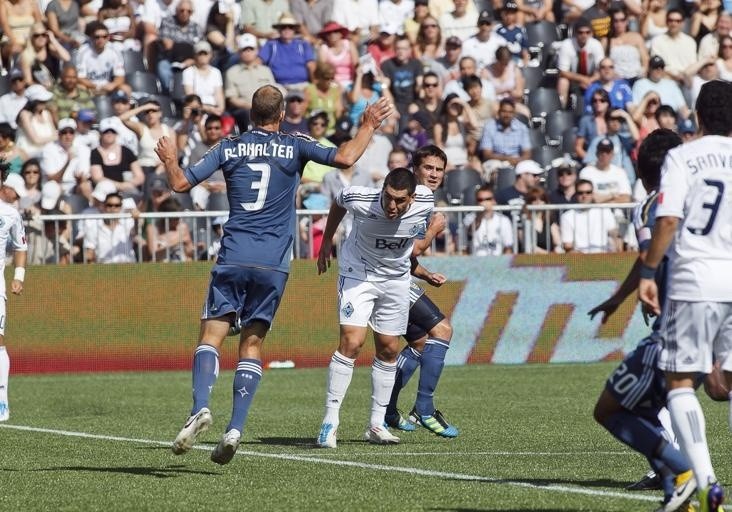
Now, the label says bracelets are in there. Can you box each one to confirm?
[13,267,25,282]
[639,263,655,279]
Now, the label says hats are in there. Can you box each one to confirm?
[4,172,27,196]
[42,182,61,211]
[378,21,396,34]
[285,87,305,104]
[480,9,492,23]
[23,86,53,102]
[92,178,116,204]
[59,92,126,135]
[192,11,349,53]
[518,158,544,179]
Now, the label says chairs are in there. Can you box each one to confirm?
[435,12,595,220]
[93,37,233,259]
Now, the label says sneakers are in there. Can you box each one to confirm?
[210,429,240,466]
[628,471,725,512]
[367,408,458,444]
[318,421,340,447]
[171,408,212,455]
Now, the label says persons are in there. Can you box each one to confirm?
[152,84,394,467]
[316,166,434,449]
[626,407,681,491]
[587,126,697,512]
[0,198,30,423]
[635,77,731,511]
[384,144,461,439]
[0,0,732,269]
